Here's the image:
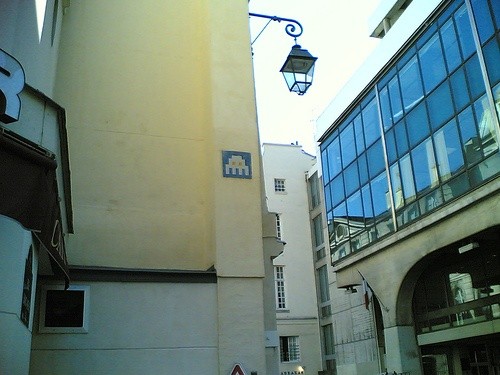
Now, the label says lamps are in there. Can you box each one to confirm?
[248,11,319,96]
